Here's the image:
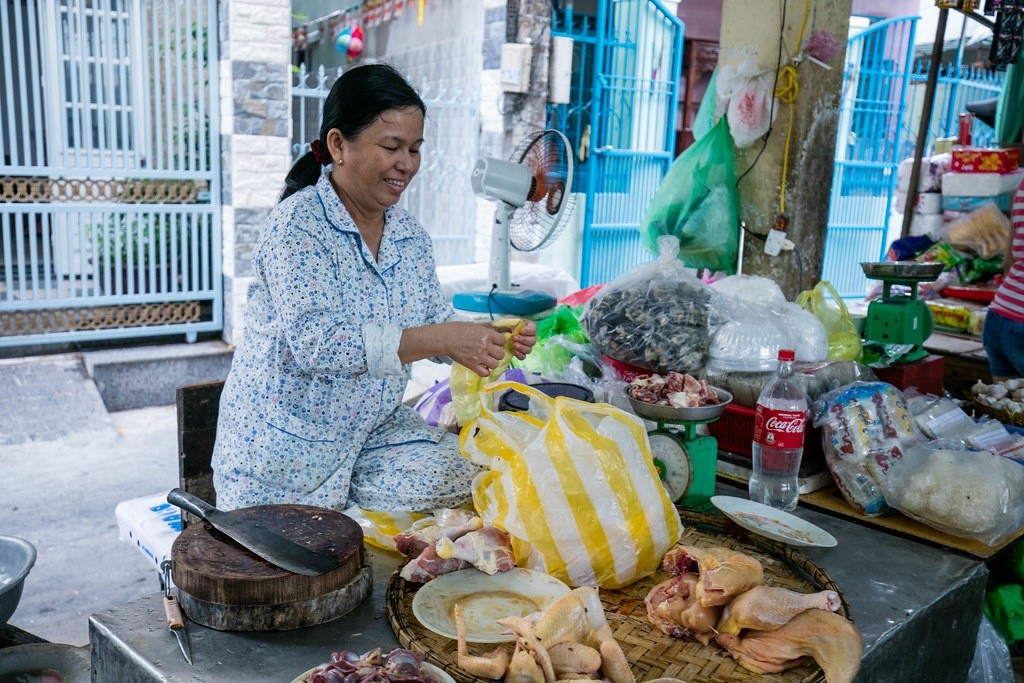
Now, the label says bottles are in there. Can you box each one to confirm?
[748,349,807,512]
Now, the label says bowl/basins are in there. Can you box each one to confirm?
[0,534,37,631]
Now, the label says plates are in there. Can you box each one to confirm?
[290,653,457,683]
[0,643,93,683]
[412,566,572,643]
[710,495,839,547]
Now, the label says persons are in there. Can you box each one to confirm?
[982,178,1024,382]
[210,65,537,516]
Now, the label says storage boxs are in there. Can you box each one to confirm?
[708,404,758,456]
[951,146,1020,174]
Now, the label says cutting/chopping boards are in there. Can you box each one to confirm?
[169,503,364,606]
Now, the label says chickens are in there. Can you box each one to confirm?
[392,516,515,583]
[453,585,635,683]
[643,542,862,683]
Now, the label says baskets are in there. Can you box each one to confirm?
[599,353,829,466]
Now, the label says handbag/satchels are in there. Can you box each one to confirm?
[412,274,716,434]
[458,381,686,590]
[642,56,779,276]
[795,280,863,362]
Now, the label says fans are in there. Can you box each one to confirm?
[453,128,580,316]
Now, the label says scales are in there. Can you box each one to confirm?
[858,261,947,363]
[624,380,733,513]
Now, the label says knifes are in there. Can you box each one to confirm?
[163,594,193,665]
[166,487,348,578]
